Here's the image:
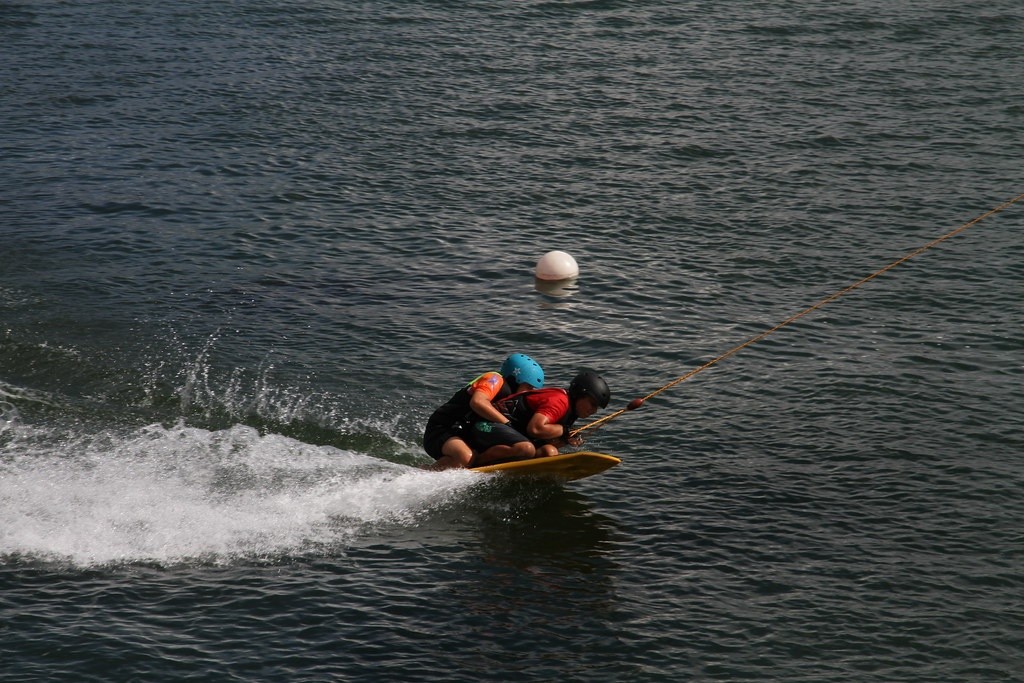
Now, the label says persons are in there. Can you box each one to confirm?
[420,353,544,471]
[462,371,611,468]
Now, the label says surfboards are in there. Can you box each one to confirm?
[467,450,622,482]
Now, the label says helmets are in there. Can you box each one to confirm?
[578,372,610,409]
[501,353,545,389]
